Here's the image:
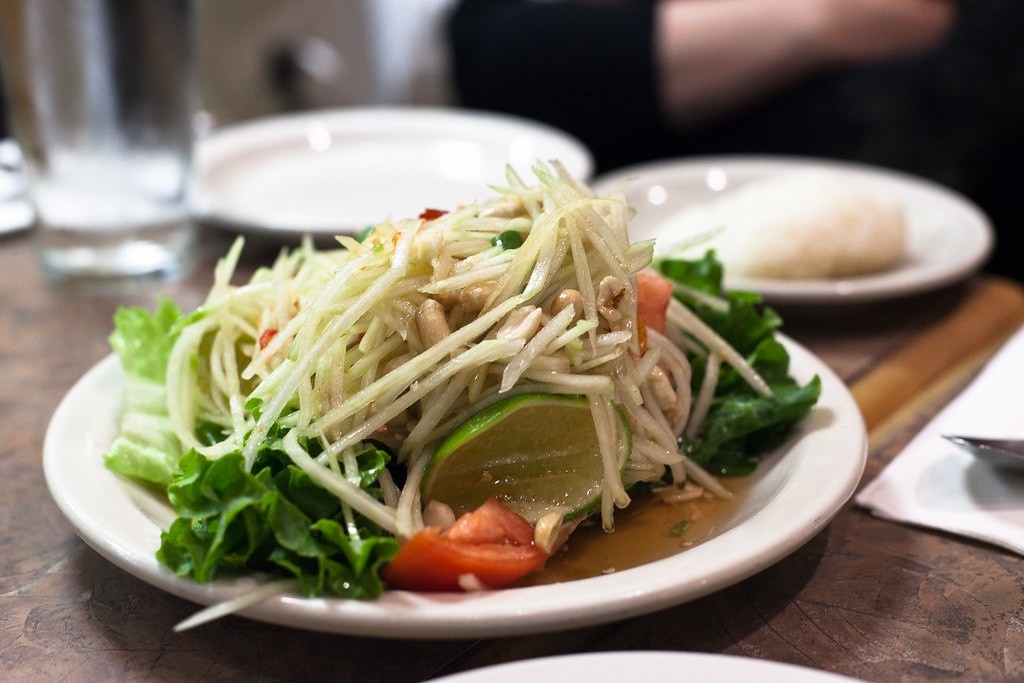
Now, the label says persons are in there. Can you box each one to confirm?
[432,0,1024,293]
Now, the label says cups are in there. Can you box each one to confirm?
[0,0,200,281]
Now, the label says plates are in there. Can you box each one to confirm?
[586,154,996,302]
[428,649,867,682]
[42,325,848,639]
[188,103,595,247]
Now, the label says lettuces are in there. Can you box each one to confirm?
[101,247,822,606]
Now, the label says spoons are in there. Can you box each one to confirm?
[941,434,1024,474]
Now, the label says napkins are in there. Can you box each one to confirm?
[851,323,1024,561]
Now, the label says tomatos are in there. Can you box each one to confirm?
[380,499,543,591]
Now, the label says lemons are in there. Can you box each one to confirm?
[420,392,633,527]
